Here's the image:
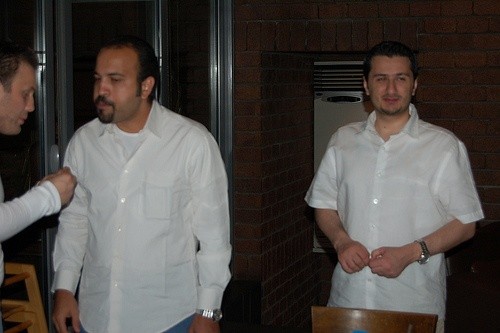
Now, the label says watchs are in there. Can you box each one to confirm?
[414,239,429,265]
[196,308,223,322]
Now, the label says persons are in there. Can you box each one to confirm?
[0,37,77,284]
[51,38,232,333]
[305,41,484,333]
